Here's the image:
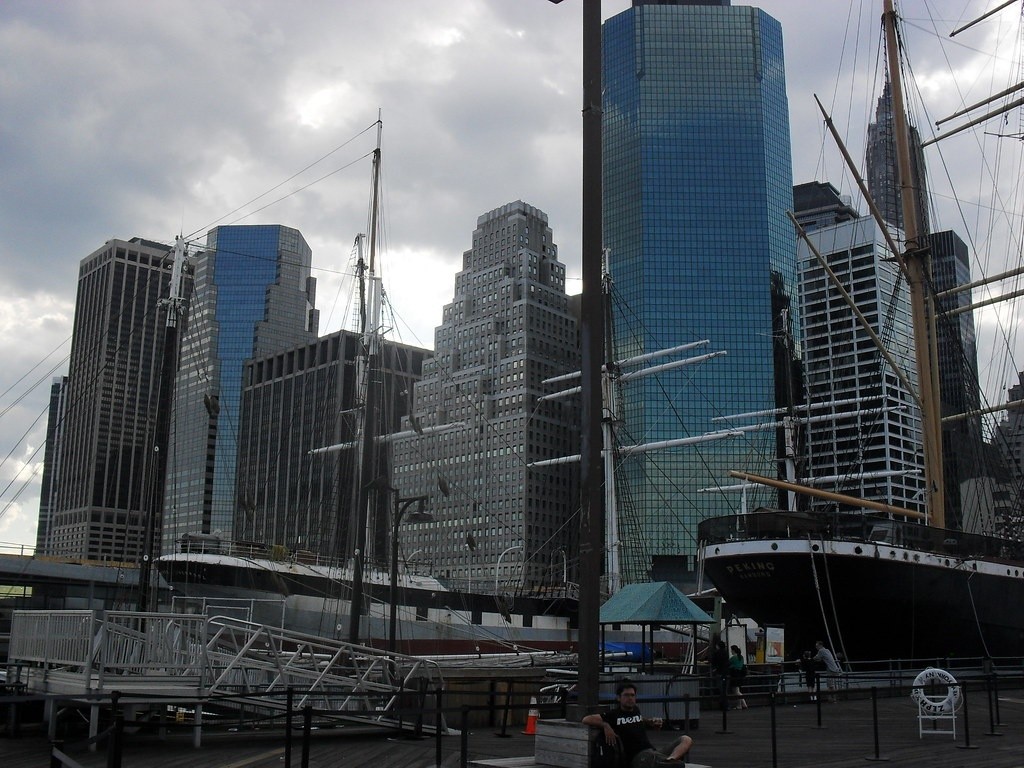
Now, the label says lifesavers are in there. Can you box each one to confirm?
[912,669,960,713]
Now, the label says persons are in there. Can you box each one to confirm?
[804,641,840,704]
[582,683,694,768]
[711,641,749,709]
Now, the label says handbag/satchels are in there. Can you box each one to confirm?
[741,665,747,678]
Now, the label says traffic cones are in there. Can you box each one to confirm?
[519,696,540,736]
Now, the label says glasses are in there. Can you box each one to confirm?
[621,692,636,697]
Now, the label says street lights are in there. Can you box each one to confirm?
[366,478,437,718]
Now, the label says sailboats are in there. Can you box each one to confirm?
[152,101,764,723]
[525,0,1024,681]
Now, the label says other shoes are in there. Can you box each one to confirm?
[743,706,748,710]
[810,696,813,700]
[733,707,742,710]
[828,698,837,703]
[814,695,817,700]
[660,757,685,768]
